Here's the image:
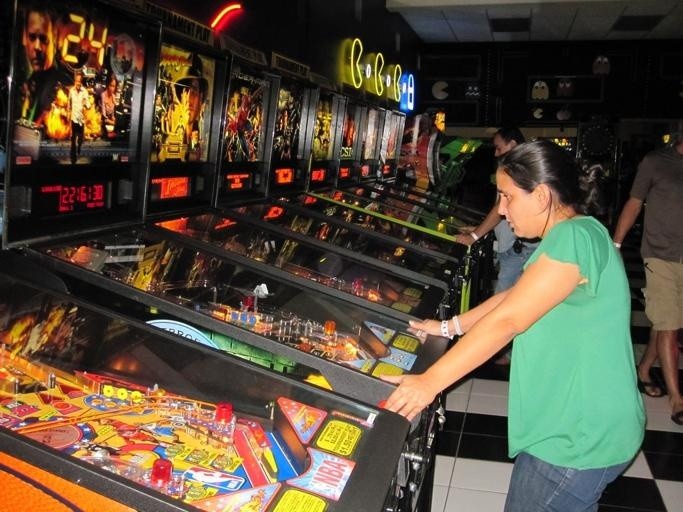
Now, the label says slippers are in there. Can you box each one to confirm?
[670,411,682,426]
[640,380,663,397]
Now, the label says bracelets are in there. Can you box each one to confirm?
[469,231,479,241]
[452,315,464,336]
[439,320,454,342]
[613,242,622,249]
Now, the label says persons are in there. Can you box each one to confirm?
[97,73,119,135]
[183,129,203,162]
[153,92,168,132]
[17,3,74,123]
[173,64,208,133]
[454,122,542,370]
[66,69,93,165]
[173,114,185,143]
[377,136,651,512]
[226,83,266,164]
[610,139,683,426]
